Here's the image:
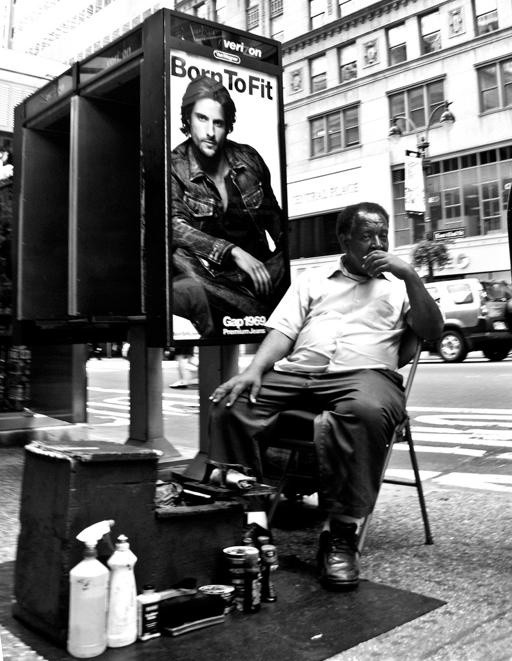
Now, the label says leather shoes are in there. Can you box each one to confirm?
[317,519,360,589]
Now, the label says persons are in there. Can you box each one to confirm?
[170,76,287,340]
[206,203,444,588]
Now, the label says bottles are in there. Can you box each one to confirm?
[135,582,162,643]
[257,535,270,547]
[259,544,280,603]
[243,537,255,548]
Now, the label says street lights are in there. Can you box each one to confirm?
[380,101,459,238]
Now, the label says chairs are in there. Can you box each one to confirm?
[241,322,434,564]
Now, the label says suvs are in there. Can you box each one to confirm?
[418,279,510,362]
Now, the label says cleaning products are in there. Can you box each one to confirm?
[67,519,115,659]
